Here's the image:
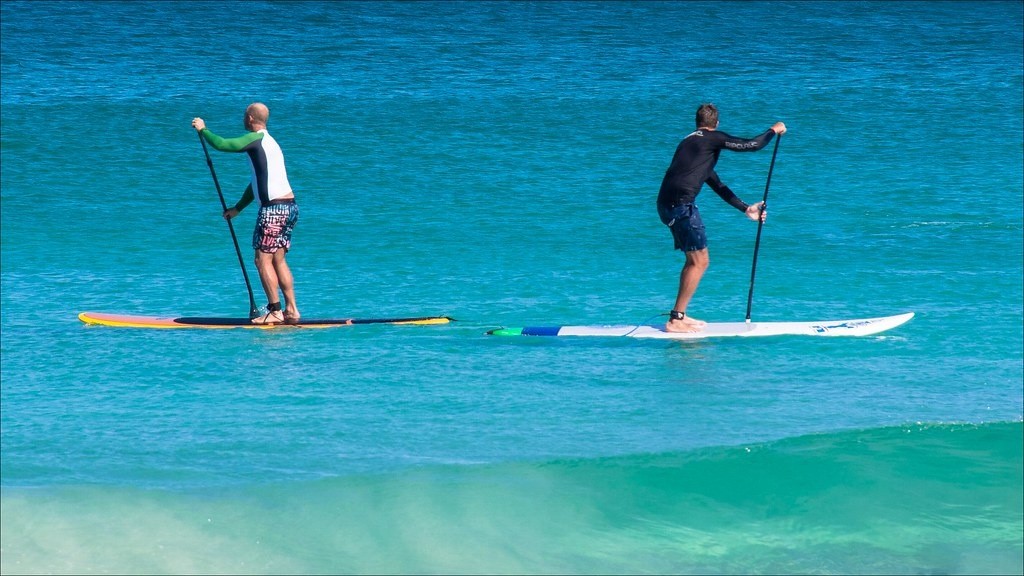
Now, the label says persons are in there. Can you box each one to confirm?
[192,103,300,323]
[657,103,786,333]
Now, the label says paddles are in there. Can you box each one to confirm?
[742,128,784,324]
[195,125,263,319]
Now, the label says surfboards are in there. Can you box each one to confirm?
[75,306,459,333]
[480,311,917,340]
